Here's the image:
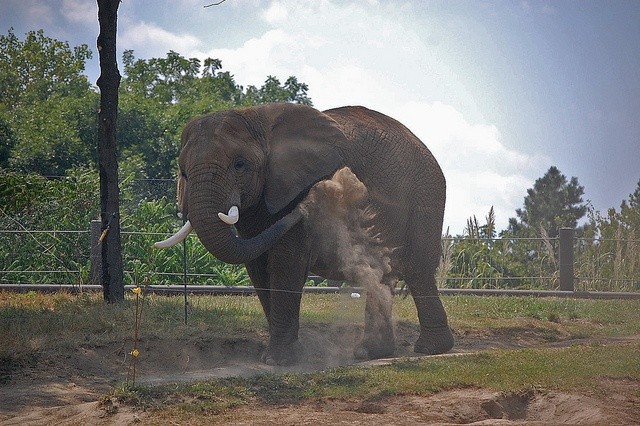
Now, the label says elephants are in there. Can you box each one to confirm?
[155,103,454,366]
